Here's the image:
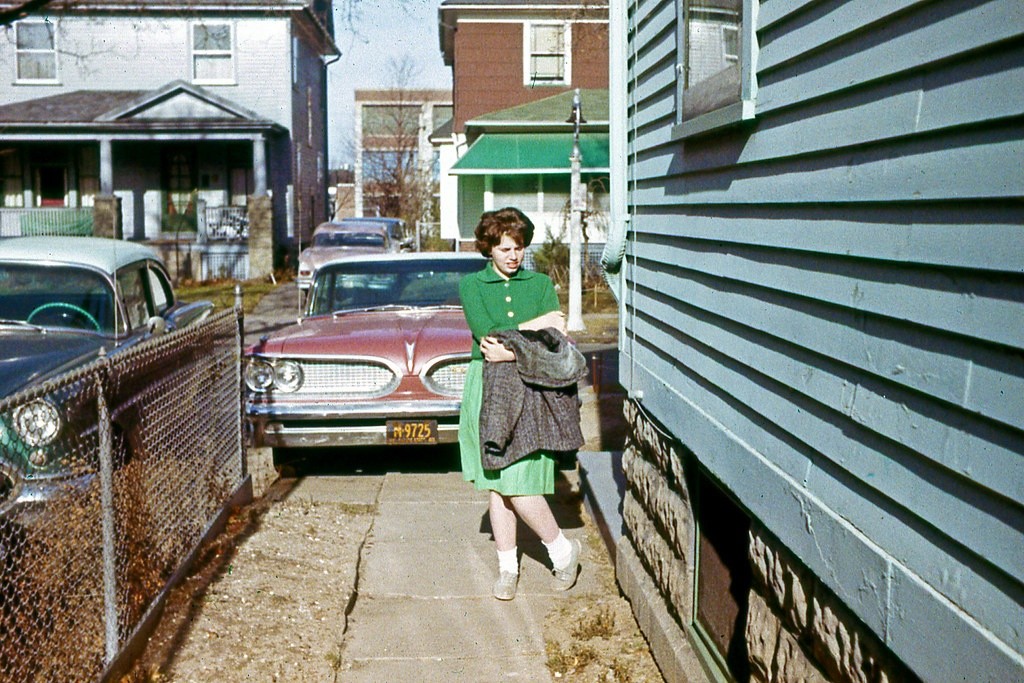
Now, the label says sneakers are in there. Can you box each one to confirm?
[551,537,581,590]
[494,570,519,599]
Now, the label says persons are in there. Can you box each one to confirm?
[460,207,582,599]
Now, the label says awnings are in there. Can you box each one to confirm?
[448,133,611,174]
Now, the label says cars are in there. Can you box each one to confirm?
[293,216,417,308]
[0,231,214,551]
[242,249,493,479]
